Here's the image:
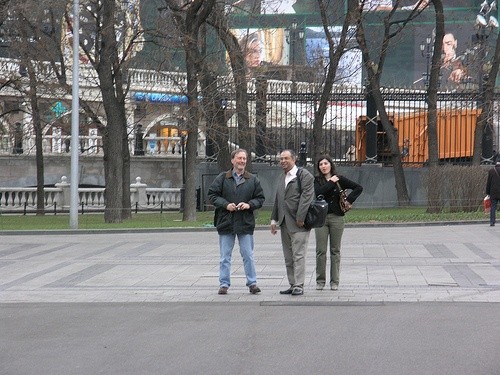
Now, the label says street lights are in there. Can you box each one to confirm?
[169,101,188,213]
[420,35,436,93]
[284,16,306,81]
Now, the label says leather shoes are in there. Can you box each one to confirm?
[279,288,304,296]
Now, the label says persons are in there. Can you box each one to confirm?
[208,148,266,295]
[314,155,362,291]
[271,149,314,295]
[485,158,500,226]
[239,33,260,68]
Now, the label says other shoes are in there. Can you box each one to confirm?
[490,222,495,226]
[317,281,338,290]
[217,287,228,294]
[249,286,261,294]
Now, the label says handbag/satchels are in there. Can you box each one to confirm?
[302,198,329,230]
[213,208,220,226]
[335,181,353,213]
[483,195,492,213]
